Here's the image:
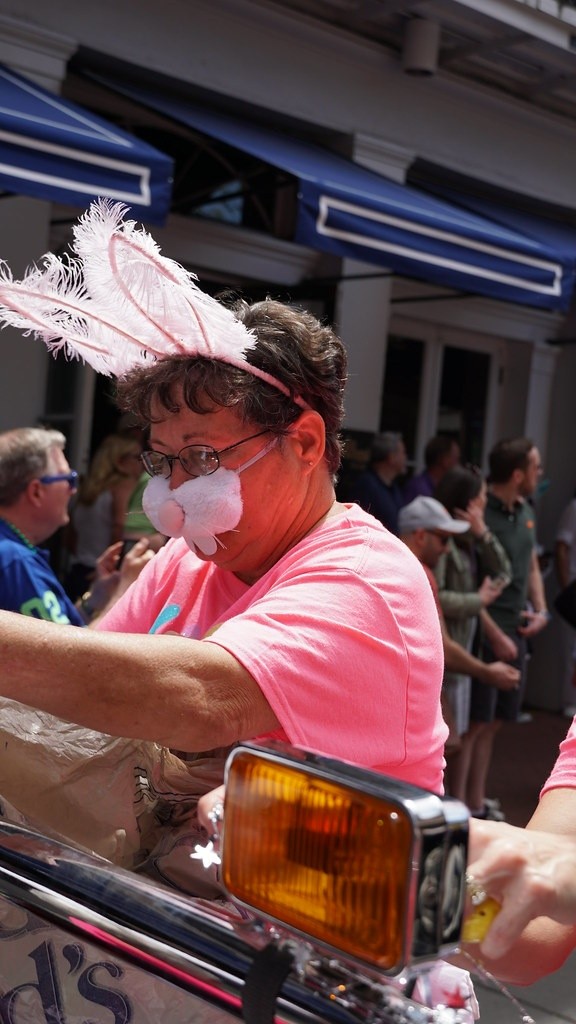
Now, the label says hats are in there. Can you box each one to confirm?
[399,494,471,533]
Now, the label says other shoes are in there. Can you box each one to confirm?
[565,706,575,719]
[470,806,507,822]
[514,712,532,723]
[482,798,502,808]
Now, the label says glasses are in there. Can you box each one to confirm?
[41,470,80,488]
[126,453,141,460]
[141,425,276,483]
[412,528,450,544]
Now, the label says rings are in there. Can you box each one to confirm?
[466,871,488,906]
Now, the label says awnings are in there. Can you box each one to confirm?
[0,61,576,309]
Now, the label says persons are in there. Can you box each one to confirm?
[198,710,575,986]
[0,295,454,797]
[0,427,157,630]
[66,415,576,820]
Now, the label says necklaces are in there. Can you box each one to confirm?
[3,517,39,553]
